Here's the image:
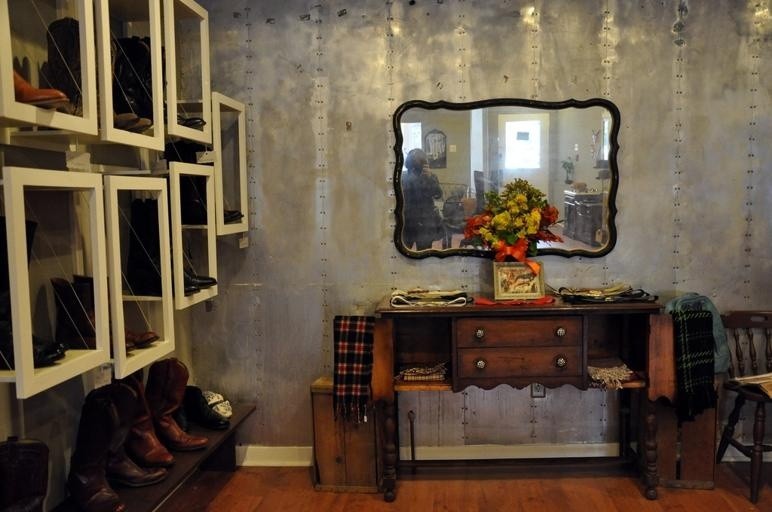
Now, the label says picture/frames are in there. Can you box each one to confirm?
[489,257,547,302]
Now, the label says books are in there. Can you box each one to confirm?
[729,373,772,398]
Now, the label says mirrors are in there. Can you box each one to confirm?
[422,128,446,170]
[389,93,624,259]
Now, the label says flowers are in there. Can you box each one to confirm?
[456,174,569,267]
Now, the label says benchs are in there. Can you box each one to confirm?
[48,398,260,511]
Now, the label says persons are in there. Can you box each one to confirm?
[401,149,443,251]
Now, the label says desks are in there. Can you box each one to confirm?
[437,206,486,251]
[368,288,669,503]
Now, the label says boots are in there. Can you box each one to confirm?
[66,358,230,512]
[127,197,217,295]
[0,217,68,369]
[166,140,243,226]
[0,435,48,512]
[51,275,159,352]
[47,17,206,135]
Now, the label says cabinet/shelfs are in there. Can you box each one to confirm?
[1,165,109,399]
[307,369,396,494]
[70,175,175,379]
[64,0,165,174]
[165,91,248,236]
[163,0,212,146]
[640,367,728,493]
[2,0,98,150]
[558,187,606,248]
[121,162,218,311]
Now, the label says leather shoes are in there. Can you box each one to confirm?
[14,69,69,108]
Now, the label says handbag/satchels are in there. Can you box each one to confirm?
[663,293,731,375]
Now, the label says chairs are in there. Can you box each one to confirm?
[711,307,771,504]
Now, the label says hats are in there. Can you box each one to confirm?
[596,170,610,179]
[593,159,609,169]
